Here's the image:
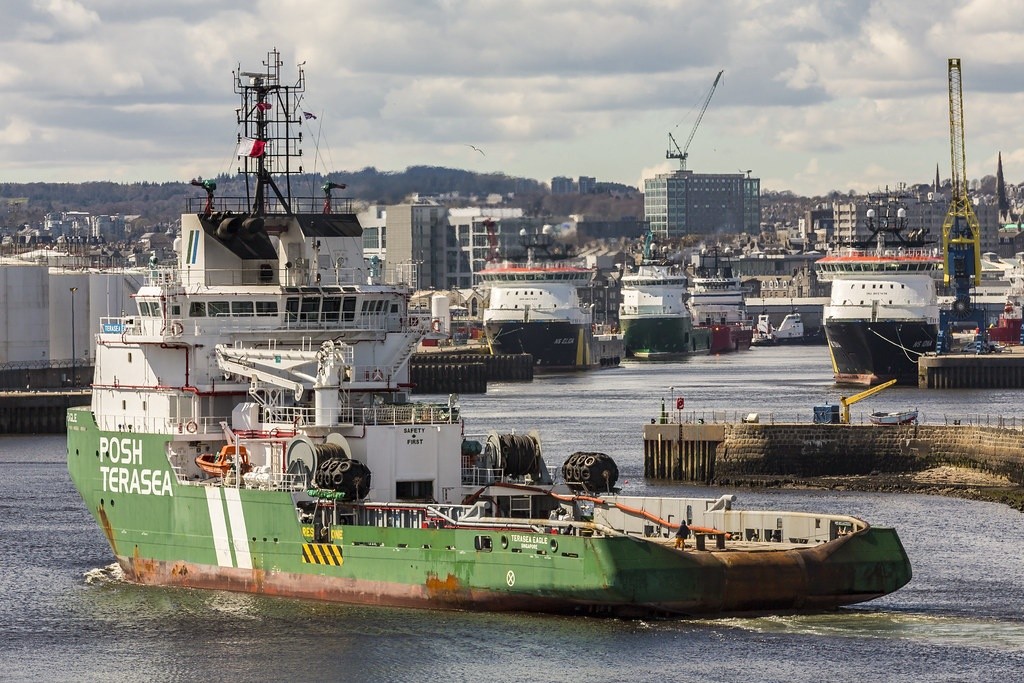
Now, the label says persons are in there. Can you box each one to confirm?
[674,520,691,551]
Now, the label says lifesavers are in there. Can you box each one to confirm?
[171,322,184,336]
[433,319,442,331]
[186,421,197,433]
[372,369,382,381]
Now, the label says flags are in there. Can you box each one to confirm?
[256,102,272,111]
[303,111,318,120]
[237,136,267,159]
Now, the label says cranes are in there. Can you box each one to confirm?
[666,67,725,170]
[937,57,989,355]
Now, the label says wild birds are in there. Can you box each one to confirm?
[464,143,487,157]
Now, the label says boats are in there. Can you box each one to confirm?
[751,295,804,346]
[820,54,1024,389]
[869,410,919,424]
[64,43,913,622]
[472,247,626,370]
[686,243,754,352]
[618,232,713,358]
